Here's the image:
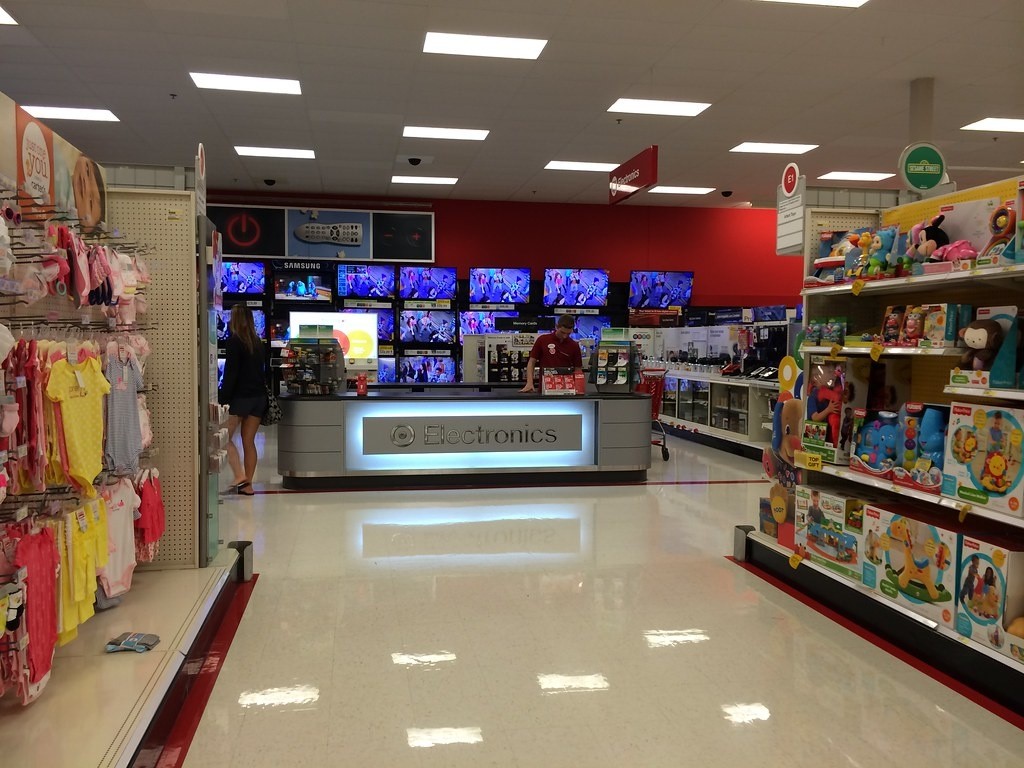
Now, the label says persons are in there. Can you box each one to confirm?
[981,411,1002,480]
[552,270,607,305]
[347,266,394,297]
[637,272,693,309]
[379,364,389,383]
[868,529,875,561]
[54,153,106,239]
[221,263,256,292]
[459,312,493,346]
[839,407,854,450]
[808,363,838,450]
[952,428,963,457]
[518,315,584,392]
[470,269,529,303]
[805,425,819,440]
[807,491,825,526]
[400,357,455,382]
[400,267,455,299]
[379,314,394,339]
[400,311,455,343]
[221,304,270,496]
[960,553,996,604]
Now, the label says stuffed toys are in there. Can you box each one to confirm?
[914,215,977,264]
[959,319,1003,373]
[870,225,896,266]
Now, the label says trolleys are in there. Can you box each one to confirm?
[634,366,671,462]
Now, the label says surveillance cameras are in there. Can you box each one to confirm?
[722,191,733,197]
[264,179,275,185]
[408,158,421,165]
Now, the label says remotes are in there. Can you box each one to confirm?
[294,223,362,245]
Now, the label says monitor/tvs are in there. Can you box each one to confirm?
[203,261,610,390]
[628,270,695,310]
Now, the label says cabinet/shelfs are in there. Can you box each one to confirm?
[745,176,1024,723]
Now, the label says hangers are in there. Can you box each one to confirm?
[8,324,141,358]
[17,450,156,531]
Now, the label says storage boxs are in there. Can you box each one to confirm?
[793,302,1024,662]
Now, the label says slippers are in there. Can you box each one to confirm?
[223,480,254,495]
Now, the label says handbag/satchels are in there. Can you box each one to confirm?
[259,384,283,426]
